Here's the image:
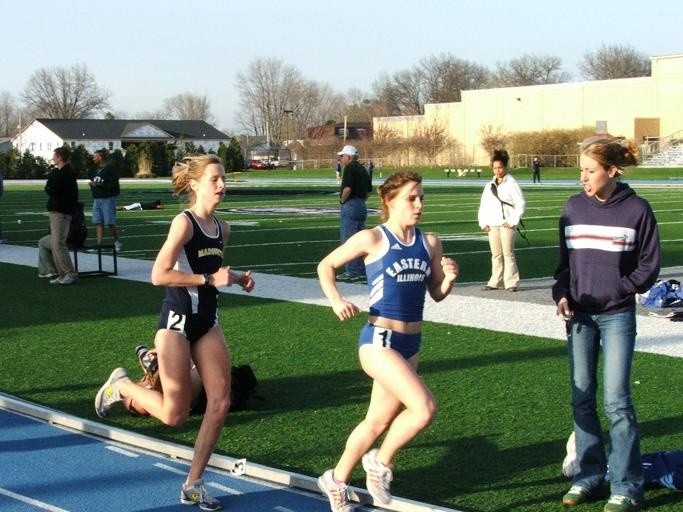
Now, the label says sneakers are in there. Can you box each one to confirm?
[38,272,79,285]
[180,478,223,510]
[136,345,153,374]
[562,485,593,507]
[336,272,366,281]
[316,469,355,512]
[361,449,393,505]
[482,286,517,292]
[113,240,122,251]
[603,494,639,512]
[93,367,131,418]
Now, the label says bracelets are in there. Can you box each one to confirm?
[204,272,209,288]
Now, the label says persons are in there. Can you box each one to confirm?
[558,429,681,493]
[367,159,374,178]
[94,152,254,512]
[476,147,525,294]
[337,145,371,281]
[334,159,342,181]
[87,147,122,254]
[36,201,87,280]
[46,147,80,287]
[550,137,660,512]
[115,198,166,212]
[313,169,462,512]
[530,156,541,183]
[121,343,257,417]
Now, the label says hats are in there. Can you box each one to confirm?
[337,145,359,155]
